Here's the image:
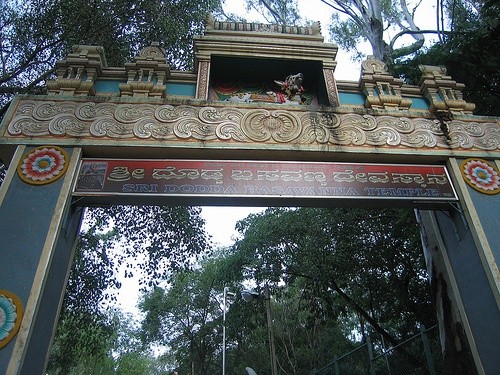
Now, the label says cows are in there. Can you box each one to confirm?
[274,73,304,95]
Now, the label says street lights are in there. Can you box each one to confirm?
[241,282,278,375]
[223,286,237,375]
